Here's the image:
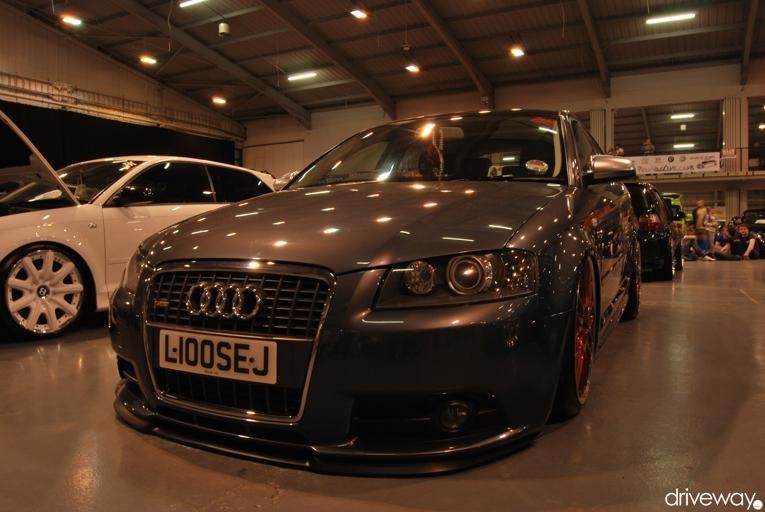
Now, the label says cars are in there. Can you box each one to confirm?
[0,108,696,484]
[740,208,765,260]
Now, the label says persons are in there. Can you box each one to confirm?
[679,206,765,261]
[692,200,712,252]
[613,142,625,157]
[639,137,656,155]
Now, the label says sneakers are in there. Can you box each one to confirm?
[702,255,716,261]
